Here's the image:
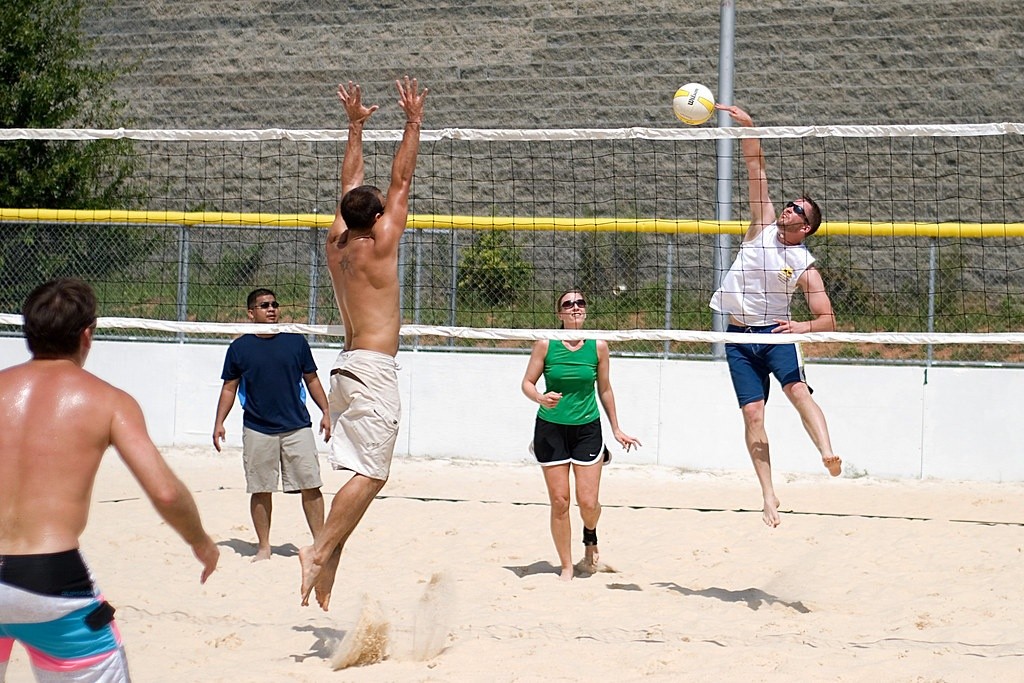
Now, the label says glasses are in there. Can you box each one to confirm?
[786,201,810,225]
[560,299,586,310]
[259,301,279,308]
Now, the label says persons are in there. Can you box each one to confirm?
[708,102,841,526]
[214,288,330,562]
[521,290,643,578]
[296,76,426,611]
[0,278,219,682]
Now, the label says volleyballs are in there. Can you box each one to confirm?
[673,81,716,126]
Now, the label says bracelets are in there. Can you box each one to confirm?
[406,121,418,123]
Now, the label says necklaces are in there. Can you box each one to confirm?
[777,233,800,246]
